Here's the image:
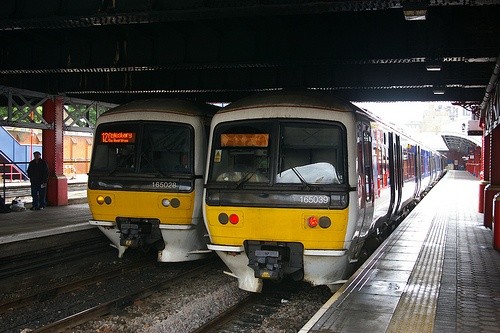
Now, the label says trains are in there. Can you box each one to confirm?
[87,99,223,268]
[202,87,450,295]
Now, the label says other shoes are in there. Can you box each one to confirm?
[39,206,43,209]
[30,206,36,209]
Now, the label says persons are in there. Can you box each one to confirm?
[175,151,190,172]
[27,151,49,210]
